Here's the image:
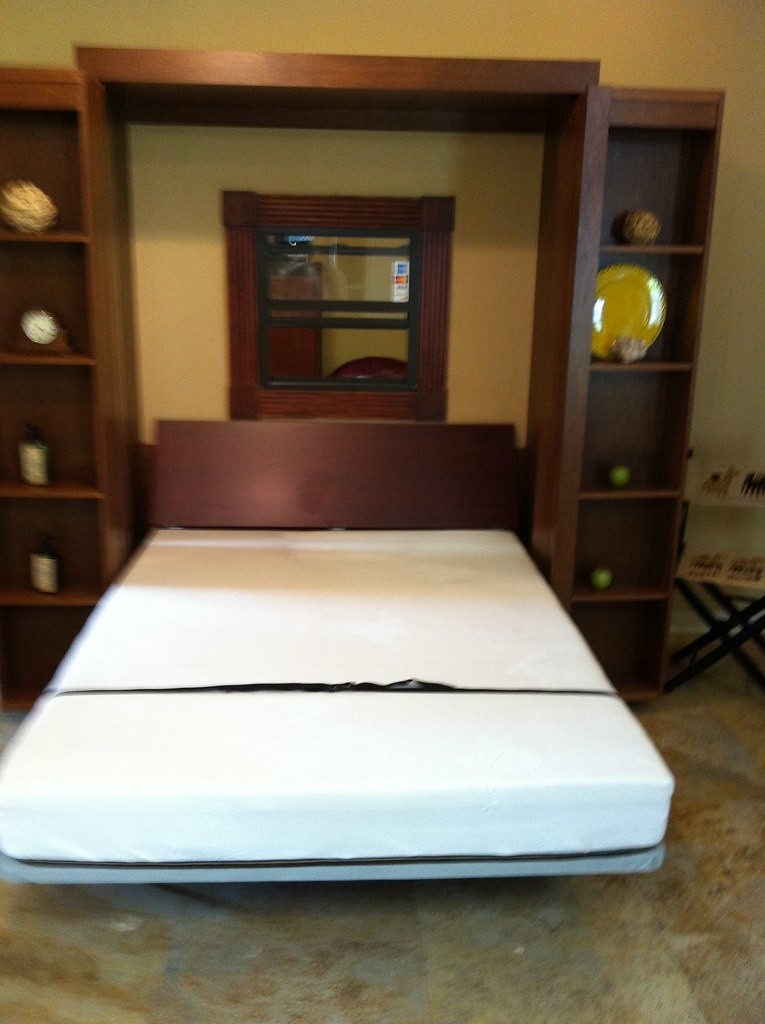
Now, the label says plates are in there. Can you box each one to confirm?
[588,262,667,359]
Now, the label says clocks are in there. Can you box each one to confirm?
[12,308,69,355]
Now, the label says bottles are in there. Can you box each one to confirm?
[27,535,64,594]
[15,425,51,486]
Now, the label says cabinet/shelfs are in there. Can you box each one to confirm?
[0,67,131,712]
[658,463,765,694]
[524,82,724,702]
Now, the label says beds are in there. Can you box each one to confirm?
[0,420,675,885]
